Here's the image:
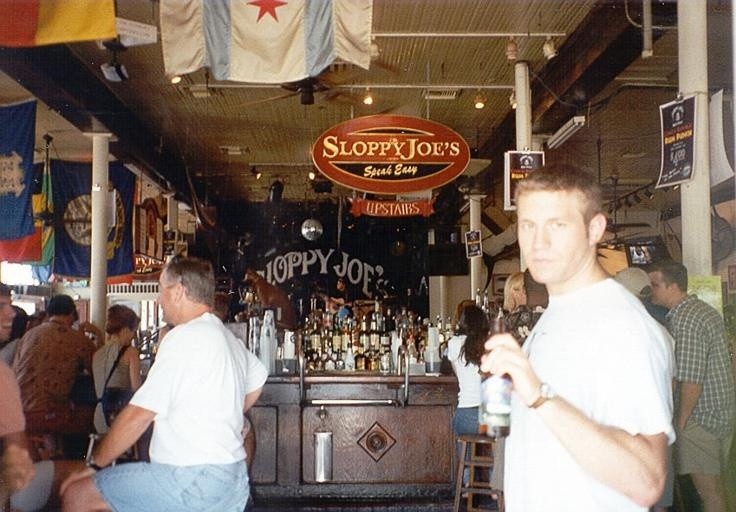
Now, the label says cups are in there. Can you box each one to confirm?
[248,310,296,375]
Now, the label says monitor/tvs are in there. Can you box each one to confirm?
[625,243,664,269]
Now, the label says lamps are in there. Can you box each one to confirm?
[504,35,519,60]
[473,88,485,111]
[268,180,283,204]
[543,35,559,61]
[544,114,587,154]
[644,187,654,200]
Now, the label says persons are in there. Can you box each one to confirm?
[0,283,173,510]
[478,163,680,511]
[614,259,736,512]
[59,253,269,510]
[446,267,549,499]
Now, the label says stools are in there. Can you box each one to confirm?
[449,433,502,511]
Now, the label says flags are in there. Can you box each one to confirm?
[46,155,139,284]
[1,0,117,48]
[30,169,59,271]
[0,163,43,263]
[0,97,38,239]
[158,1,376,85]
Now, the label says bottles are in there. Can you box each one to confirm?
[304,296,453,375]
[479,319,512,438]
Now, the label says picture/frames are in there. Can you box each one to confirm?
[492,273,511,295]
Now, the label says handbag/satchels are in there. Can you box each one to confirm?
[101,387,134,415]
